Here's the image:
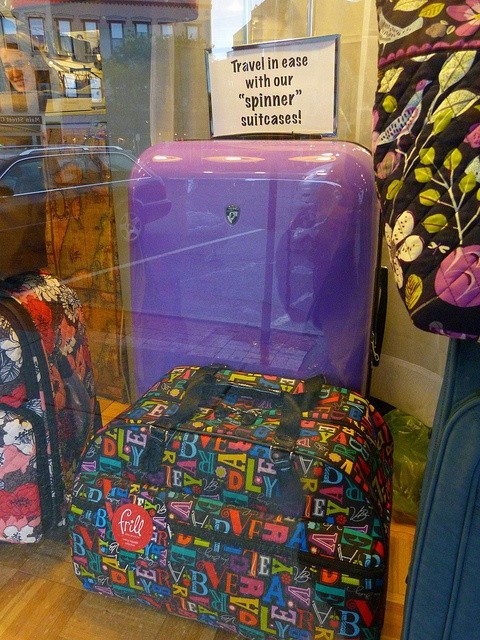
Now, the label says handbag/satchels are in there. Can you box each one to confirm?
[67,365,393,639]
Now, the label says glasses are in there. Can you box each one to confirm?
[2,58,36,73]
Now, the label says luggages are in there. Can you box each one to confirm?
[402,341,480,637]
[0,271,101,545]
[127,2,386,406]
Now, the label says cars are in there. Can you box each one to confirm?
[1,146,172,281]
